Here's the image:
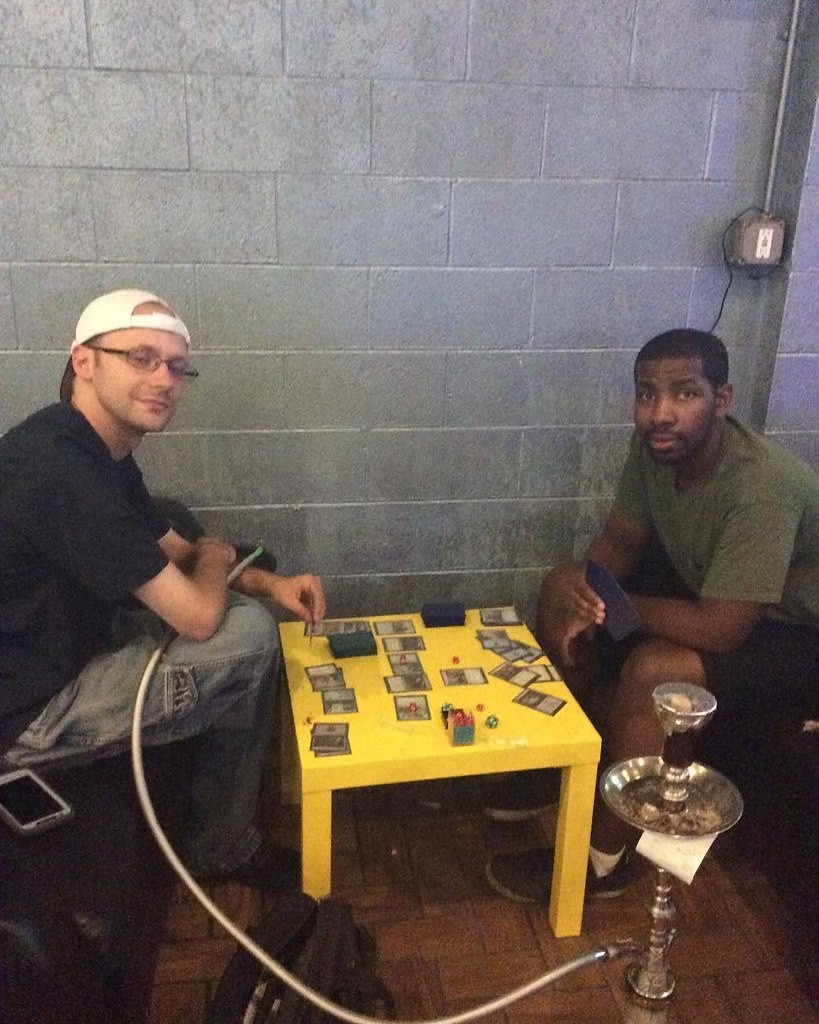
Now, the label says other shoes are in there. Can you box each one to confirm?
[193,842,301,888]
[479,768,559,821]
[485,845,628,904]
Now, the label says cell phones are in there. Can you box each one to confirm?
[0,768,77,839]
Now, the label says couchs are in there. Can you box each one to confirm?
[0,498,277,1024]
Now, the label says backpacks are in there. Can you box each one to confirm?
[211,892,366,1024]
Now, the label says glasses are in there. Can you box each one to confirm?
[84,346,200,386]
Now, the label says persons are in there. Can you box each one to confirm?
[478,326,819,905]
[0,287,326,891]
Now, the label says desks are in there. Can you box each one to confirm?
[278,609,602,939]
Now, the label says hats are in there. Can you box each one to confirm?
[59,289,191,403]
